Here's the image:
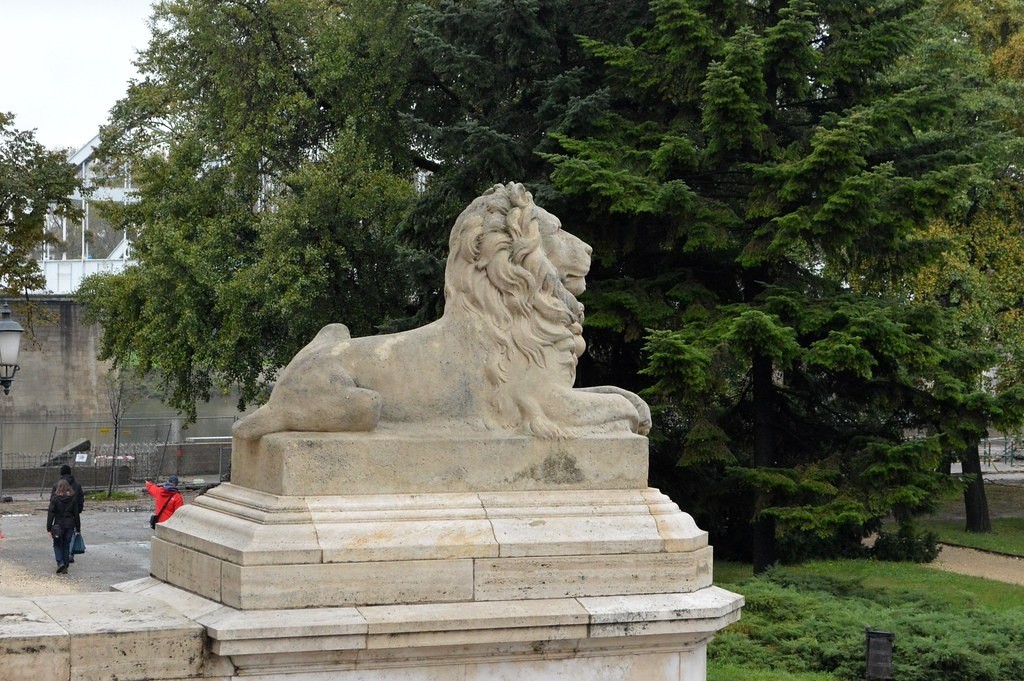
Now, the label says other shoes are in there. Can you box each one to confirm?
[69,555,75,563]
[55,562,66,573]
[63,567,68,574]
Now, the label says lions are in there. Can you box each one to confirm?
[230,180,652,443]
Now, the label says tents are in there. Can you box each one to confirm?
[980,435,1017,467]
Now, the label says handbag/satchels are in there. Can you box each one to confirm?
[72,534,87,554]
[149,515,159,530]
[50,524,62,540]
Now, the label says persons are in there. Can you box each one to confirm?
[50,465,85,563]
[47,479,81,574]
[144,474,183,523]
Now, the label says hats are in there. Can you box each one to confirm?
[169,476,178,482]
